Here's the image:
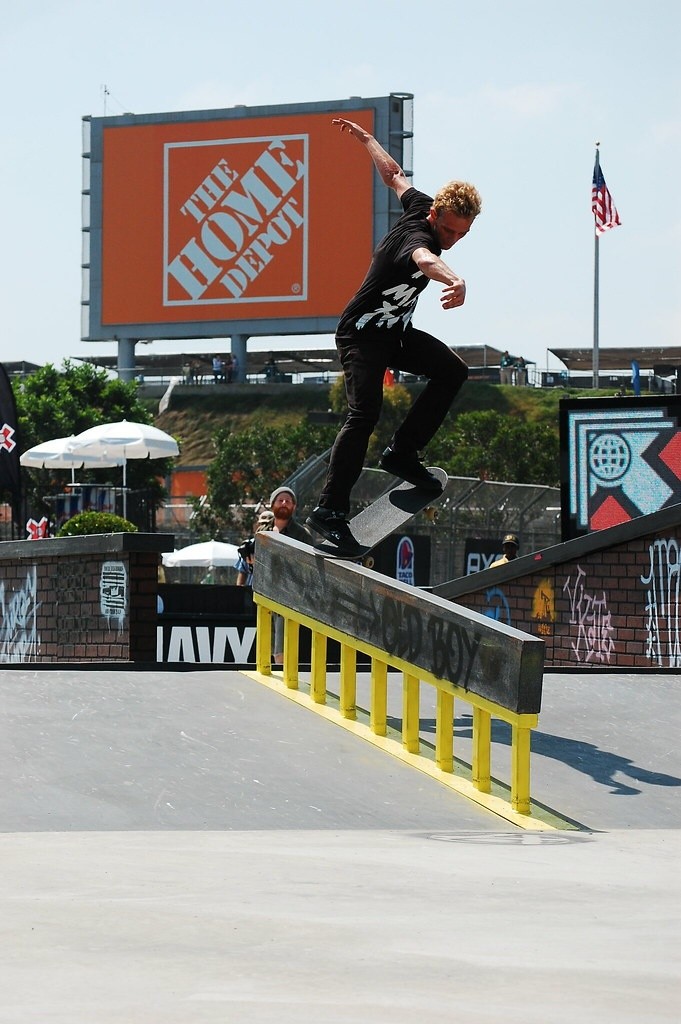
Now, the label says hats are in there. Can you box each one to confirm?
[270,487,297,507]
[502,534,520,549]
[258,511,274,523]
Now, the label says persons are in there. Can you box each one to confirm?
[490,533,519,569]
[213,352,238,384]
[501,350,526,368]
[233,487,312,586]
[305,117,482,556]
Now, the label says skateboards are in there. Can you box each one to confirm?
[311,465,449,560]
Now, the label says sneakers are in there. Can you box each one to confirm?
[379,446,442,490]
[306,507,361,552]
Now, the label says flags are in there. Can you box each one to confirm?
[591,162,623,236]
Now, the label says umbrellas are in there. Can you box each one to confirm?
[61,418,180,520]
[161,539,240,584]
[19,434,126,495]
